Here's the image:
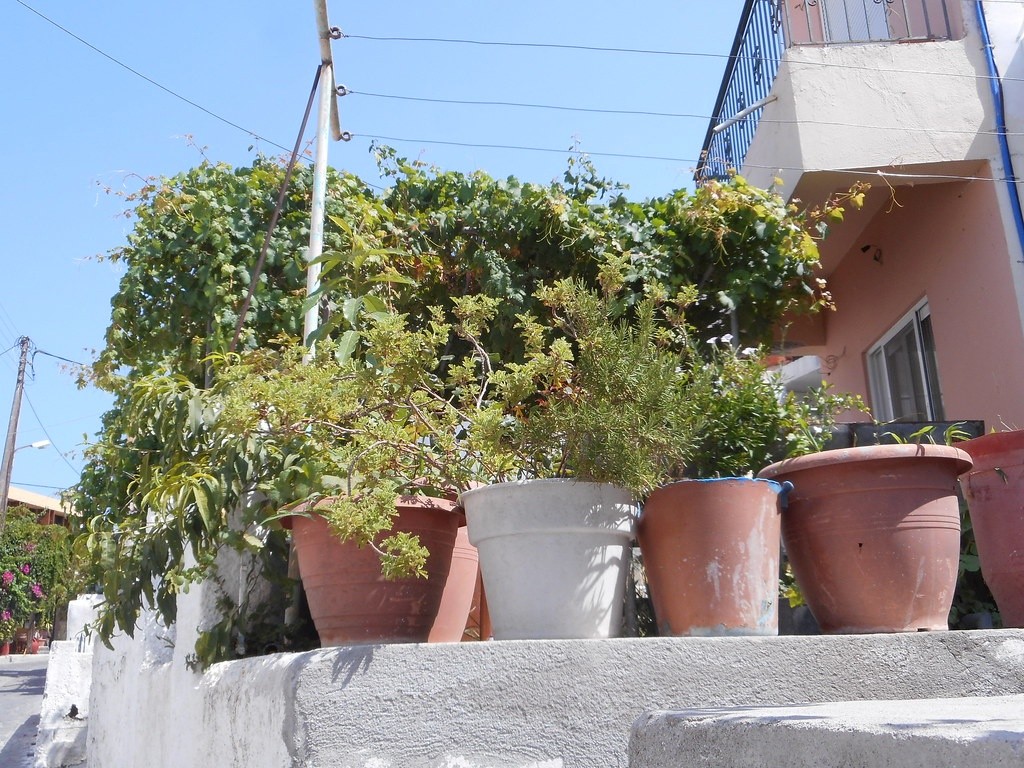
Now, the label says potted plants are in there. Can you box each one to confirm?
[211,291,575,643]
[456,279,712,639]
[632,332,795,635]
[758,379,974,635]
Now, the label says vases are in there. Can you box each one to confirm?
[948,430,1024,628]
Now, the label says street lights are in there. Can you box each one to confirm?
[0,439,51,544]
